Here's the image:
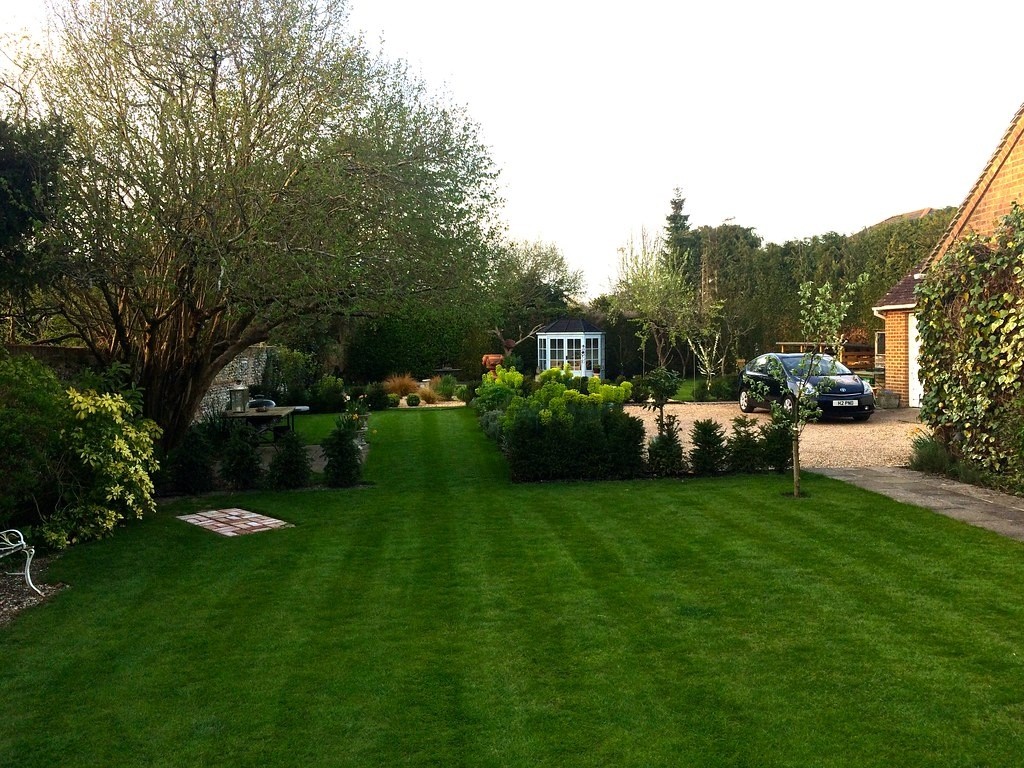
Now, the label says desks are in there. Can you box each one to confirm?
[221,407,296,445]
[432,368,462,375]
[287,406,310,432]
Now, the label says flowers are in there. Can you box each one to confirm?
[341,391,371,415]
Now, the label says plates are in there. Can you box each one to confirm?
[256,409,268,412]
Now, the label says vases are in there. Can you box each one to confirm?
[346,413,372,446]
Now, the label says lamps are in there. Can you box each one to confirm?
[229,383,249,414]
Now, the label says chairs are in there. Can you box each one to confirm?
[249,400,276,408]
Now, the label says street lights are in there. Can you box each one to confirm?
[637,345,645,390]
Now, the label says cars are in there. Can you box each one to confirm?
[737,352,877,427]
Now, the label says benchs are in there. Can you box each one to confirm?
[0,529,45,598]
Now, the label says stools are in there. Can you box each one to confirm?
[422,379,431,387]
